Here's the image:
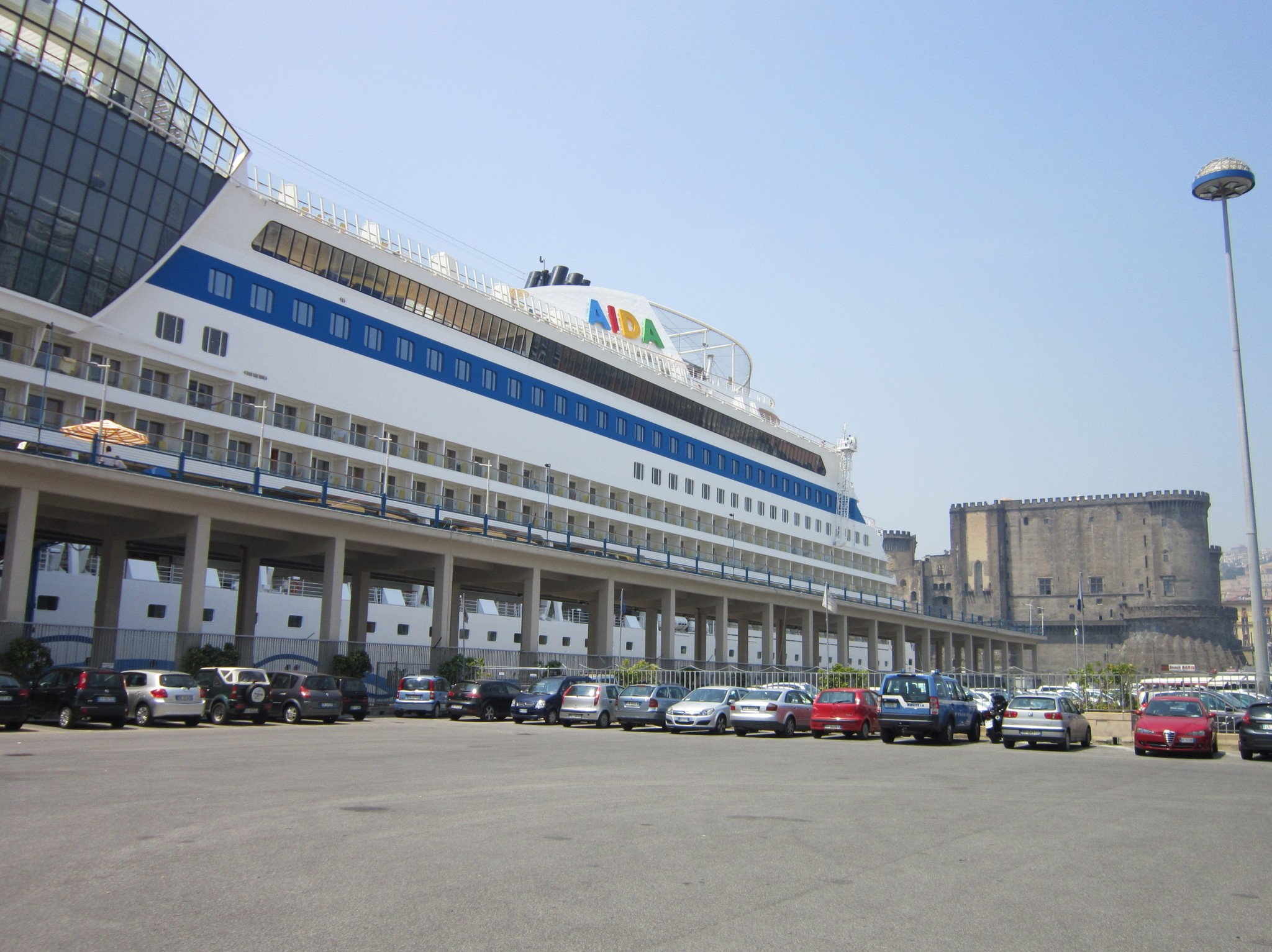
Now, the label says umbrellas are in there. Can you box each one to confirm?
[60,419,151,457]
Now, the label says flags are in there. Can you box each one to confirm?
[619,590,626,615]
[1076,575,1085,616]
[462,593,469,624]
[822,584,838,614]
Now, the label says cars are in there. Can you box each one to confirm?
[0,671,1272,758]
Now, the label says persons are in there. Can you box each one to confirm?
[114,455,127,469]
[1134,680,1158,702]
[99,446,116,467]
[231,576,240,591]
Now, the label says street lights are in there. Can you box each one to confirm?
[1190,160,1272,690]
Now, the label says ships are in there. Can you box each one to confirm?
[3,0,928,673]
